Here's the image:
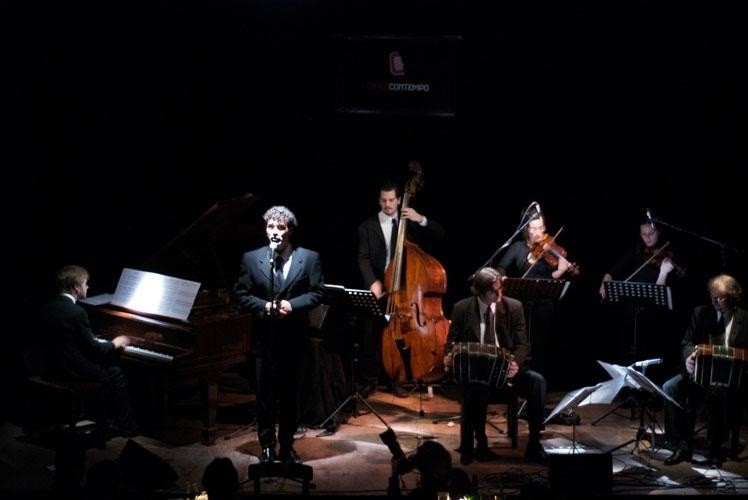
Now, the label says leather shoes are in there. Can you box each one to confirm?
[460,448,476,466]
[524,443,549,464]
[361,384,376,395]
[697,457,722,467]
[386,380,409,397]
[664,447,692,465]
[262,446,275,462]
[278,449,301,464]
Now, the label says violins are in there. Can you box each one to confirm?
[521,235,579,272]
[647,247,685,274]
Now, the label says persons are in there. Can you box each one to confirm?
[203,458,239,500]
[46,430,93,500]
[351,185,445,397]
[599,221,695,317]
[662,275,746,465]
[495,208,580,307]
[38,267,130,439]
[385,442,470,497]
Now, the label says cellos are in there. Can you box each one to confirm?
[382,160,450,386]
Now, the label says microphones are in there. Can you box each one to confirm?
[268,242,278,263]
[635,358,663,367]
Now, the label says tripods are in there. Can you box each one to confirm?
[237,274,319,490]
[502,318,572,418]
[319,328,391,429]
[591,319,673,432]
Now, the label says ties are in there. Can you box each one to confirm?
[719,312,726,345]
[390,219,398,259]
[484,305,495,346]
[275,256,285,287]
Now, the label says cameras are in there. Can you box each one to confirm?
[379,429,418,476]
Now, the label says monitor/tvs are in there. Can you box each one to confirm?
[334,35,462,117]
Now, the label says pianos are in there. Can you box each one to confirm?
[78,293,251,447]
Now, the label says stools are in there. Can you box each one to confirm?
[27,377,105,450]
[685,390,744,459]
[455,392,519,454]
